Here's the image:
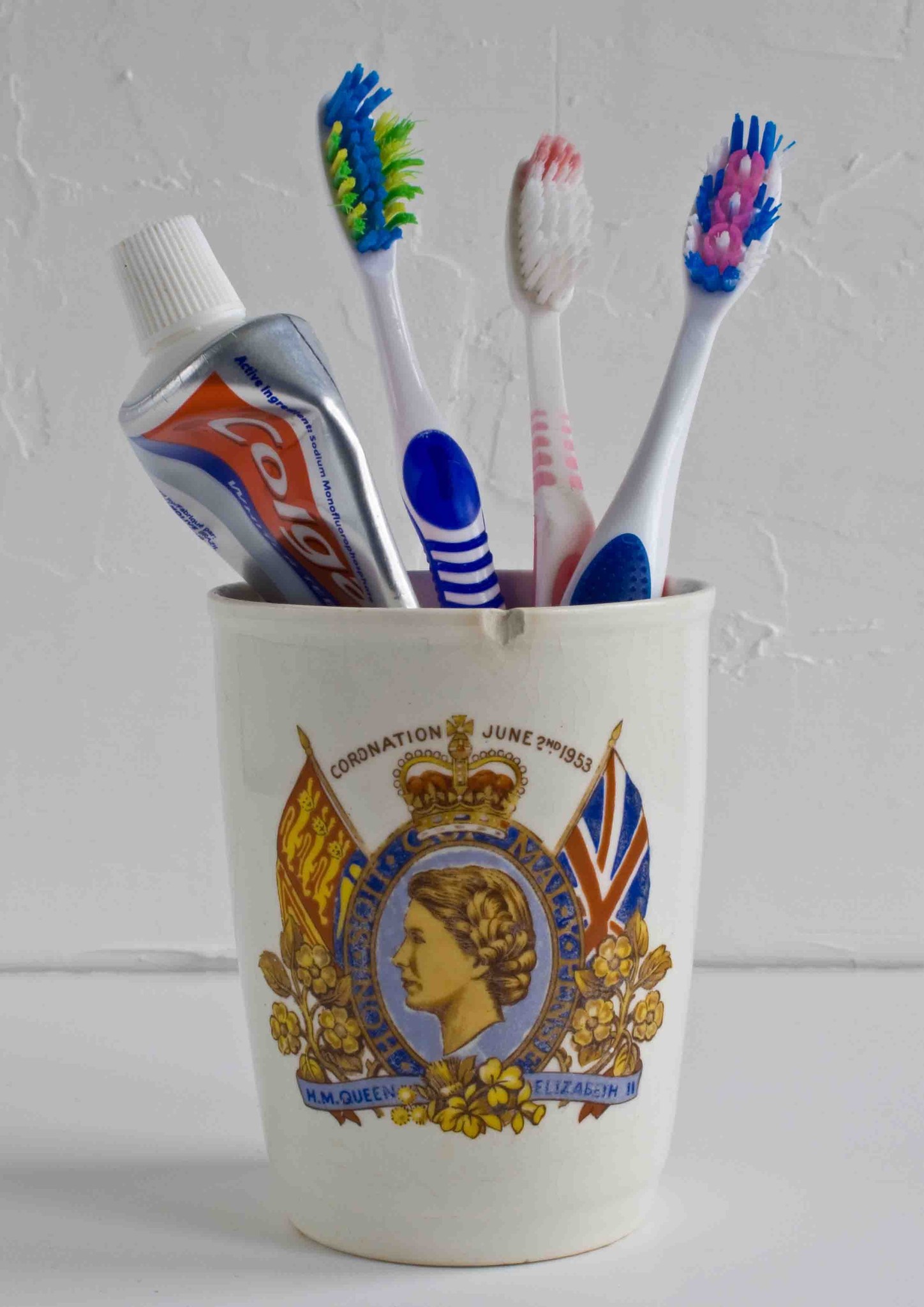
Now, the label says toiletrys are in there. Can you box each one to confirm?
[109,210,422,613]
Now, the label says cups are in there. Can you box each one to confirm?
[198,571,721,1277]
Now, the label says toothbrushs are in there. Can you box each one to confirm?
[314,60,510,606]
[556,115,791,611]
[500,138,609,611]
